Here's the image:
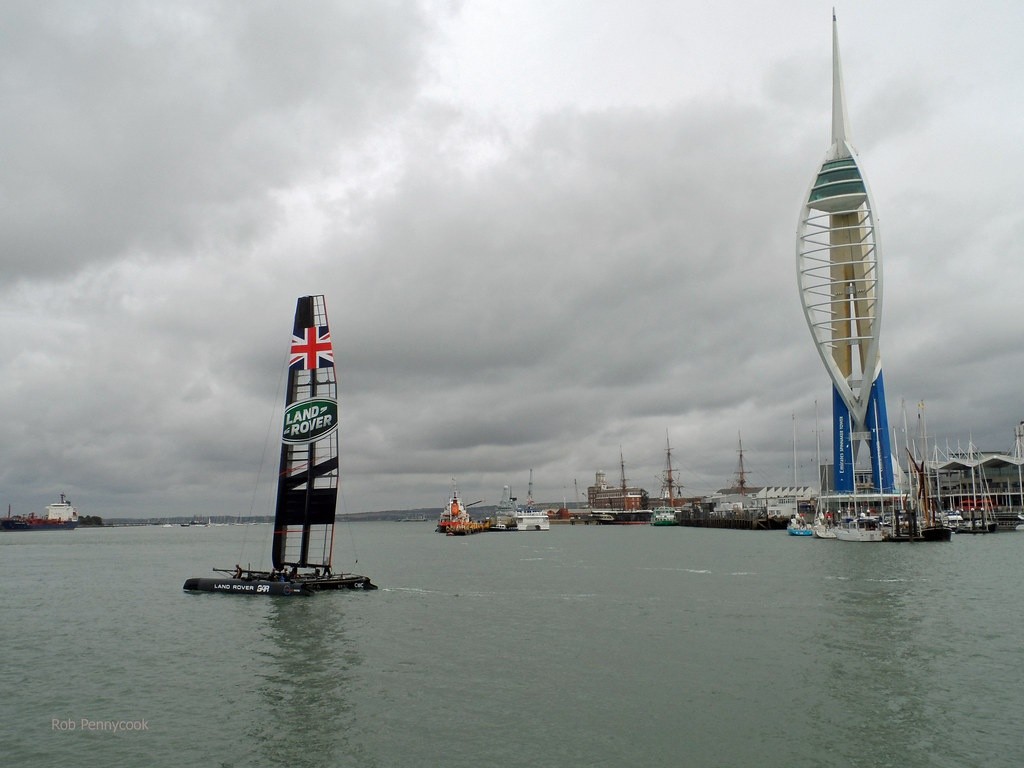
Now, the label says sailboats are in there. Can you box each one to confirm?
[590,430,783,526]
[161,513,270,528]
[182,295,379,599]
[1014,424,1024,521]
[787,411,814,537]
[809,395,999,543]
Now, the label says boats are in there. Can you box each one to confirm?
[489,519,507,529]
[511,470,551,531]
[434,476,472,534]
